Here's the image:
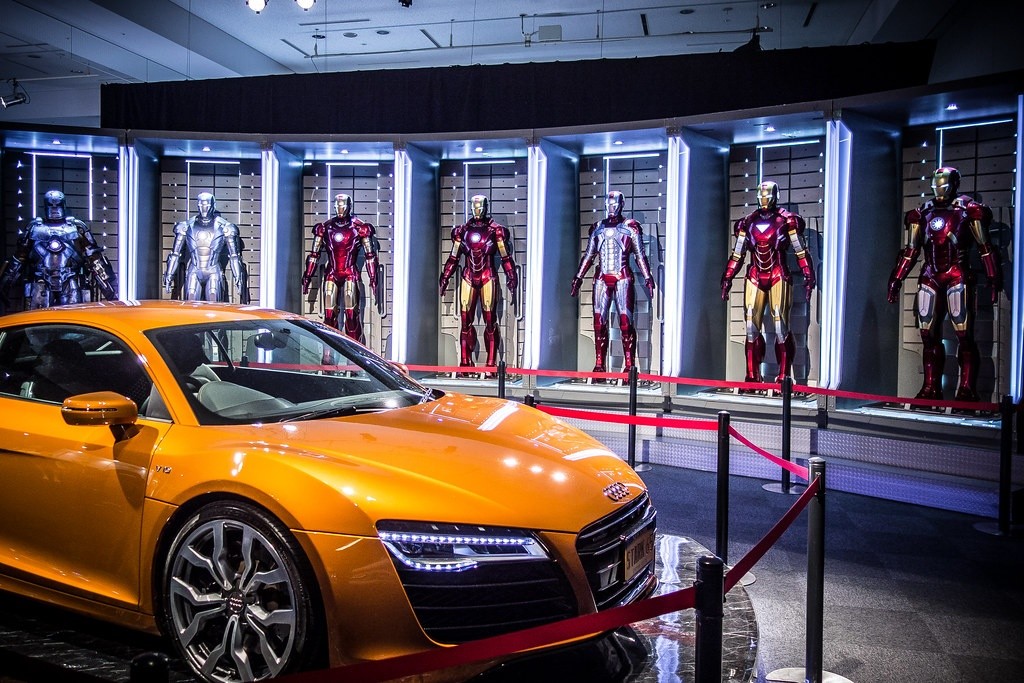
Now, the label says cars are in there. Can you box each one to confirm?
[0,295,660,683]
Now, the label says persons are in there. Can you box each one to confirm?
[721,181,816,398]
[439,195,518,379]
[0,190,115,311]
[885,167,1004,413]
[164,192,251,362]
[301,195,386,377]
[570,193,655,385]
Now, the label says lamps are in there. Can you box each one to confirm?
[246,0,270,14]
[294,0,316,11]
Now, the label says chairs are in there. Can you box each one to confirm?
[19,333,223,412]
